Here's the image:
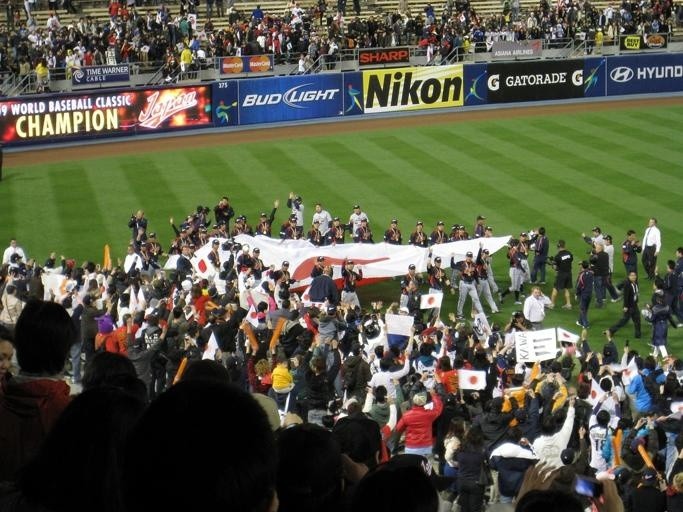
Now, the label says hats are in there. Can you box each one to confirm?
[327,304,337,311]
[412,390,429,407]
[10,252,22,263]
[251,392,282,435]
[140,197,494,272]
[560,447,574,465]
[509,225,612,269]
[388,453,458,495]
[281,411,304,429]
[514,310,524,319]
[640,466,657,487]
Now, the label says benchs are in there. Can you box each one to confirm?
[0,1,625,71]
